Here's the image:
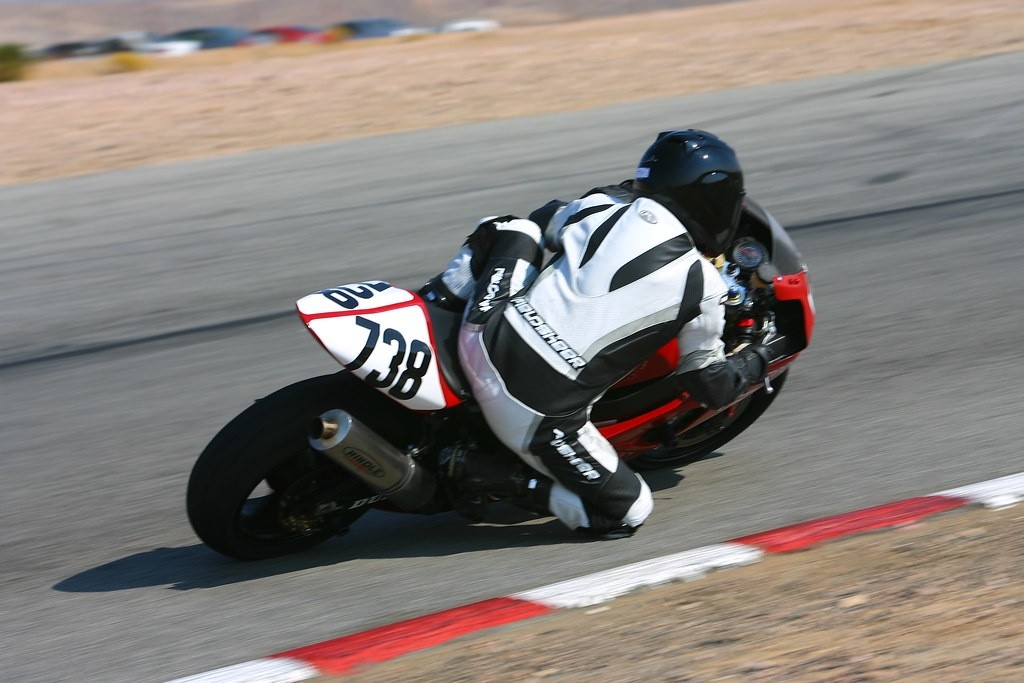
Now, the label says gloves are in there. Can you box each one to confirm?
[756,330,791,365]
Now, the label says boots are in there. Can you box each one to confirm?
[449,454,553,526]
[416,271,467,311]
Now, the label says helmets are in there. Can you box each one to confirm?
[633,130,744,250]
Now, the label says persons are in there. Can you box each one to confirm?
[419,130,781,542]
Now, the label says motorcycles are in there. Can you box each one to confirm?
[185,197,816,562]
